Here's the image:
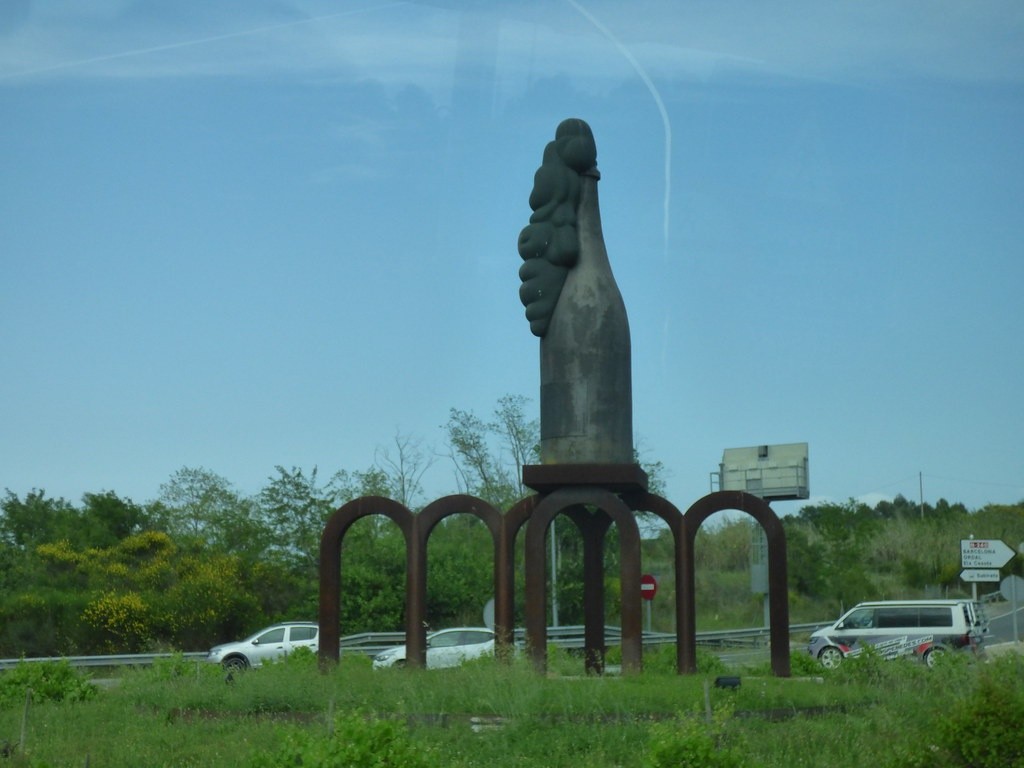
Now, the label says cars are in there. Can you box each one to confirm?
[373,623,495,671]
[209,623,321,676]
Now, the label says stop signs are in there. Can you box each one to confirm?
[640,574,658,600]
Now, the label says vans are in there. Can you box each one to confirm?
[801,598,986,669]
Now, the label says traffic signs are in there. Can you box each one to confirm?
[959,570,1001,583]
[959,539,1016,570]
